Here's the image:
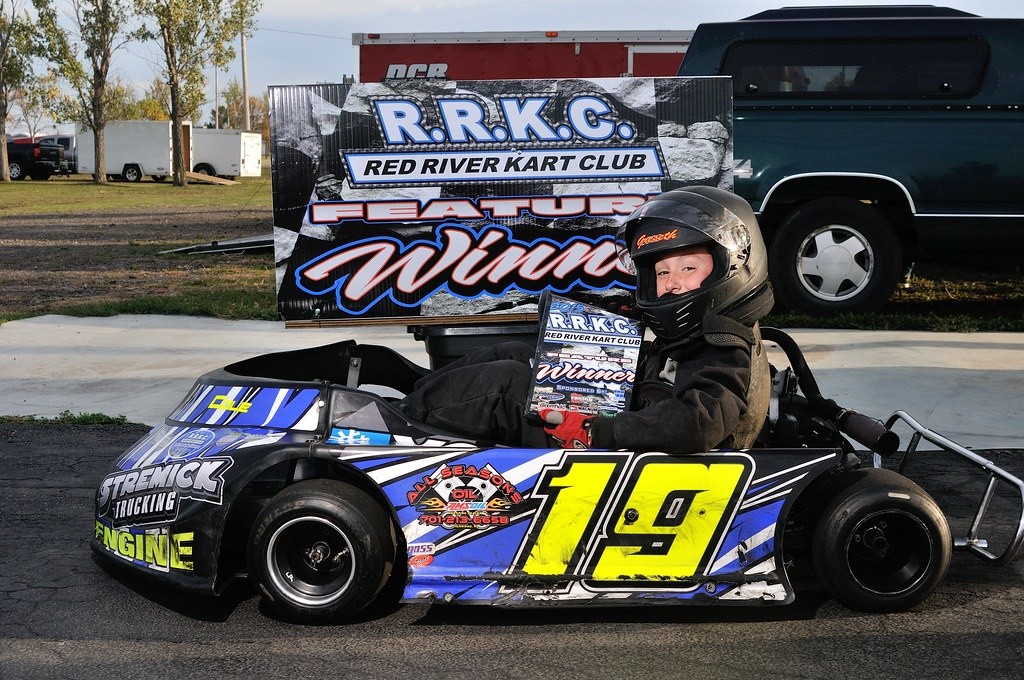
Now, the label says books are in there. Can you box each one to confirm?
[524,295,646,422]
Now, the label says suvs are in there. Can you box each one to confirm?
[674,1,1024,321]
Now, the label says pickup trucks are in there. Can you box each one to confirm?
[0,141,65,181]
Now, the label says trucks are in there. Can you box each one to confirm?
[37,134,76,173]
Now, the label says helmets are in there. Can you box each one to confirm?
[615,185,774,340]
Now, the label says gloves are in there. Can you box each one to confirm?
[600,295,639,320]
[539,410,598,449]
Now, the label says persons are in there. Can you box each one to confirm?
[390,185,774,454]
